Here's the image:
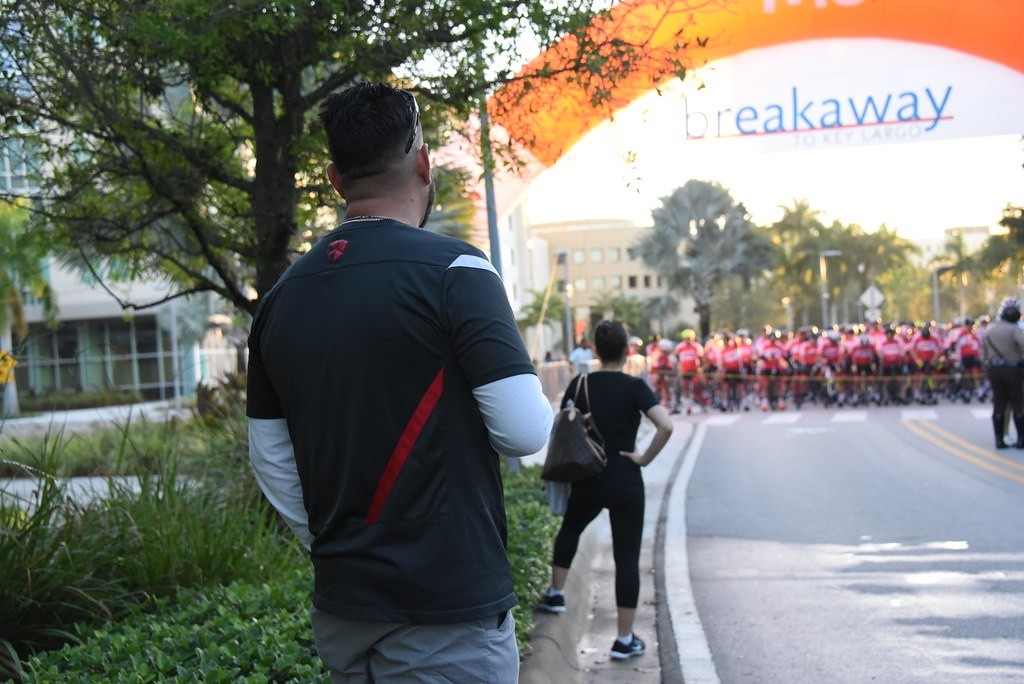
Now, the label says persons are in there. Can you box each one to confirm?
[980,306,1024,449]
[569,296,1024,445]
[246,81,555,684]
[537,320,673,659]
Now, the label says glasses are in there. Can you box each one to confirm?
[400,88,420,154]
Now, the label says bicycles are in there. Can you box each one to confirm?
[652,354,992,414]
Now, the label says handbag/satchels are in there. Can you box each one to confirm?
[541,371,609,484]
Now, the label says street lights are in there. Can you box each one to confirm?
[931,262,953,325]
[819,248,842,329]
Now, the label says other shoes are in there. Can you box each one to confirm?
[664,389,990,415]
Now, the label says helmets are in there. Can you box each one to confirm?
[625,314,991,350]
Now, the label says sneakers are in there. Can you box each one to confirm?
[537,587,565,612]
[610,635,646,659]
